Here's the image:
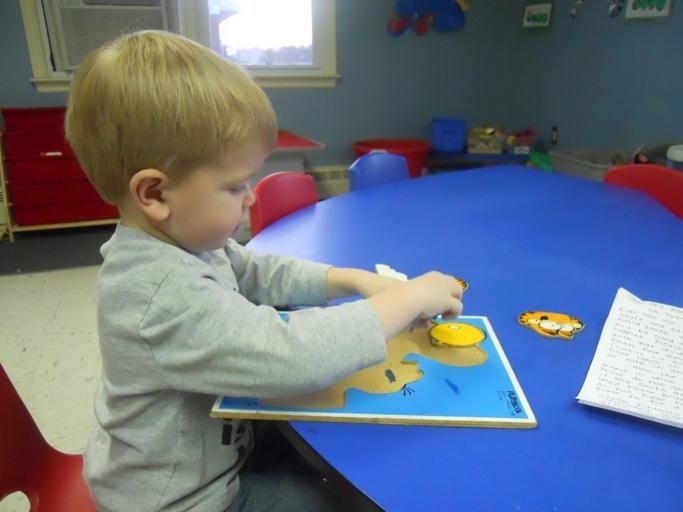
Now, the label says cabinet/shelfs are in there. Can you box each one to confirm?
[0,107,121,242]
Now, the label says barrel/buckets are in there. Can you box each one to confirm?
[351,137,433,178]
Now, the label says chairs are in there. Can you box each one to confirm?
[604,165,683,219]
[248,153,410,237]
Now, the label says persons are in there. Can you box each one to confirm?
[60,25,467,512]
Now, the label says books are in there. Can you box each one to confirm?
[571,286,683,432]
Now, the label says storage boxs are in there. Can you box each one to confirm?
[430,118,538,155]
[548,148,633,180]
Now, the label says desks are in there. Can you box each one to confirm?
[230,130,326,242]
[242,163,682,511]
